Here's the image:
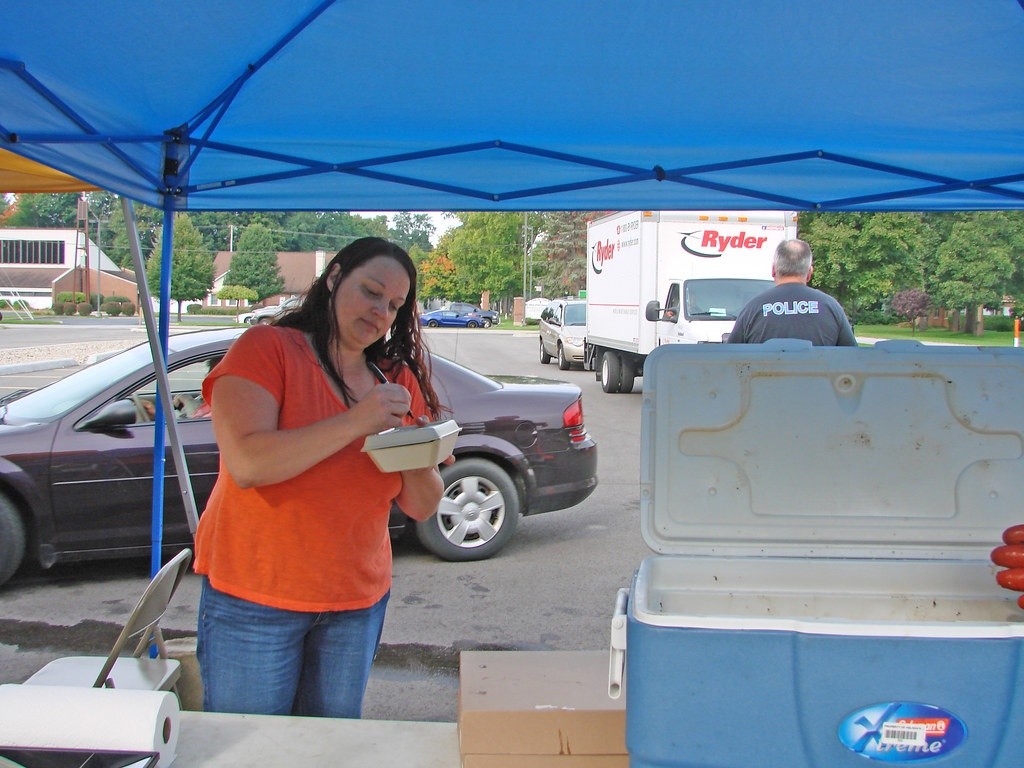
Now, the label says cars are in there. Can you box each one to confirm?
[1,327,600,591]
[418,309,486,329]
[235,295,306,326]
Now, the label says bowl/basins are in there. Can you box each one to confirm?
[360,420,463,474]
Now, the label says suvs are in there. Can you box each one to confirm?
[538,294,586,371]
[448,302,501,328]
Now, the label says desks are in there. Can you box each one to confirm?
[167,711,462,768]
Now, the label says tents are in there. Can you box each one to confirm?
[0,0,1024,657]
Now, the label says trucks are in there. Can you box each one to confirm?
[582,210,798,393]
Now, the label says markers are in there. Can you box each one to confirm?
[366,360,414,420]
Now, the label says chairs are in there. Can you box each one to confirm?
[22,549,192,710]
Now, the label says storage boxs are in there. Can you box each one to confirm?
[606,338,1024,768]
[457,650,632,768]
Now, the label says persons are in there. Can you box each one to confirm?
[668,291,701,316]
[142,357,223,420]
[194,237,456,719]
[728,239,857,346]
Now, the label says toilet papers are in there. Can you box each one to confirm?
[1,680,182,766]
